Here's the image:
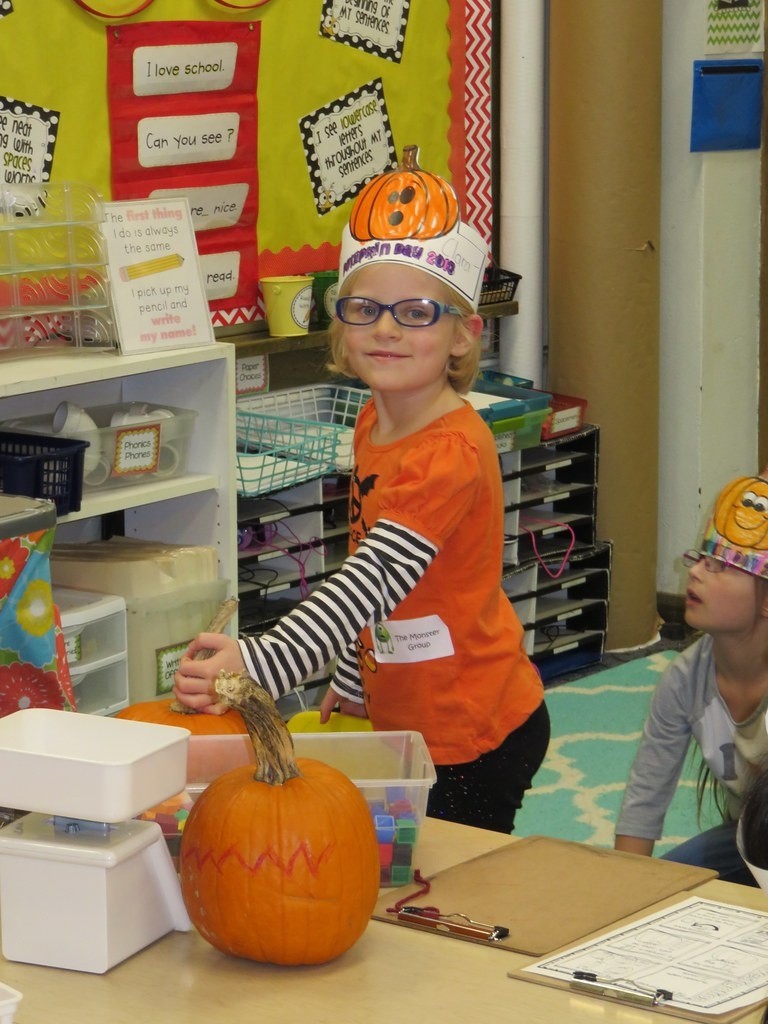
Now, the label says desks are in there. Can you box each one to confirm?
[0,818,766,1024]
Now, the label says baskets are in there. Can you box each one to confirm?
[235,383,374,474]
[236,410,348,497]
[0,431,89,516]
[478,267,522,307]
[478,370,532,392]
[525,388,588,441]
[0,981,23,1024]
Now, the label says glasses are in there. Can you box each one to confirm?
[682,549,739,574]
[335,297,464,328]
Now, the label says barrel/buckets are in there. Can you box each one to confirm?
[258,276,314,336]
[306,270,339,329]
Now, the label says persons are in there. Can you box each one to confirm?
[615,476,768,894]
[172,218,550,836]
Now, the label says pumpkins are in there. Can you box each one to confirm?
[176,670,380,965]
[106,597,257,780]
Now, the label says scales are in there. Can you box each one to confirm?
[0,708,192,975]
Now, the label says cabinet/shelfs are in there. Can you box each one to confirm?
[0,339,239,715]
[0,182,117,353]
[239,427,614,712]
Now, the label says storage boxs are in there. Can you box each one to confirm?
[0,707,190,824]
[140,730,438,886]
[463,370,589,453]
[44,586,129,716]
[2,400,197,496]
[45,580,230,704]
